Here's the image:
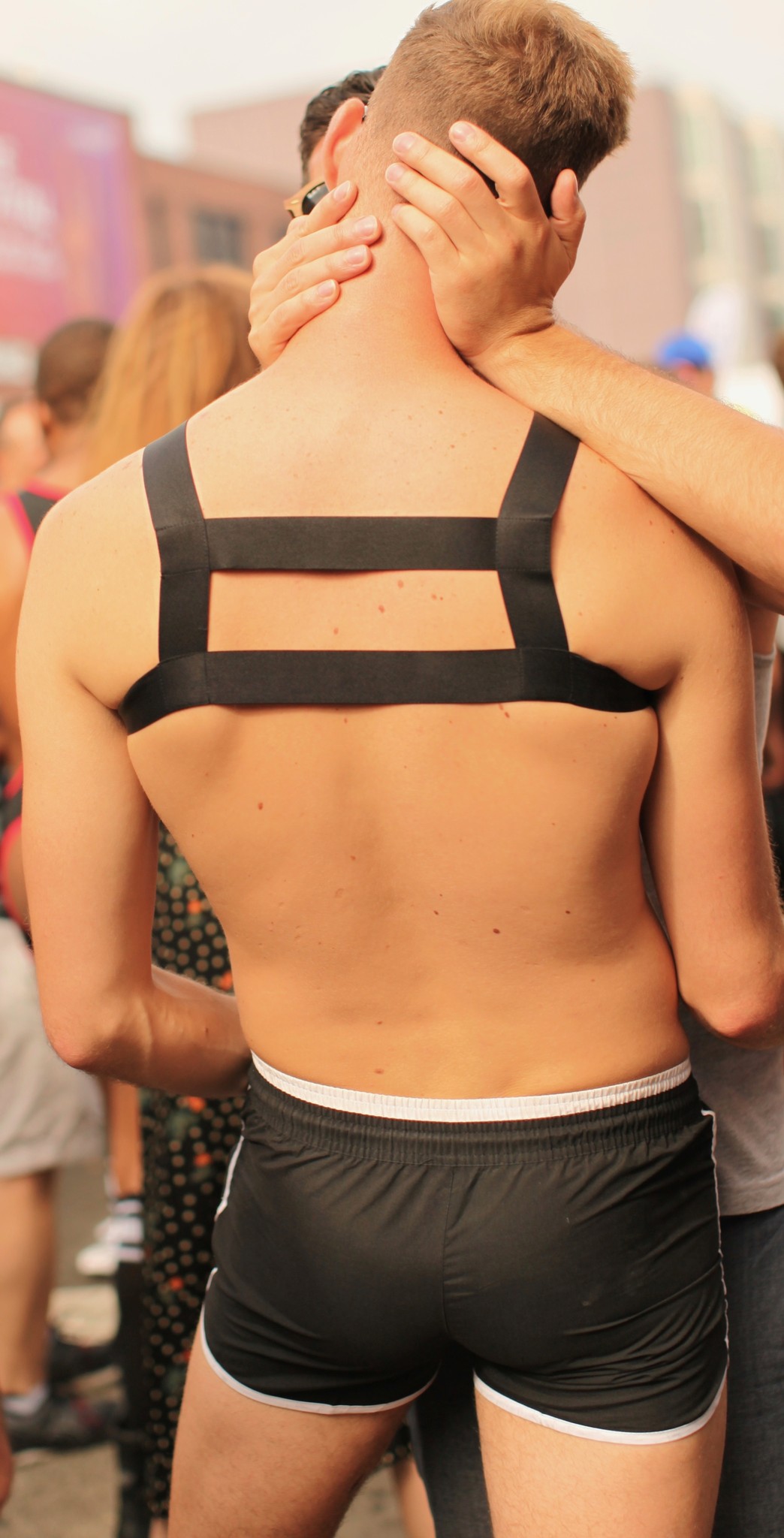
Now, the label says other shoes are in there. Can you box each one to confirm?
[3,1392,126,1449]
[49,1339,111,1384]
[81,1195,147,1276]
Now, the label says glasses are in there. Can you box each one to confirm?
[284,178,329,218]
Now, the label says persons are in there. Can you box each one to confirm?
[19,1,784,1537]
[2,88,784,1538]
